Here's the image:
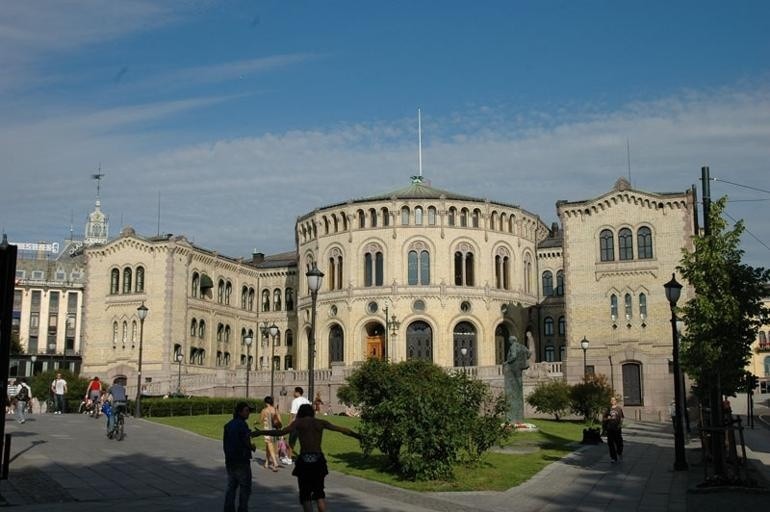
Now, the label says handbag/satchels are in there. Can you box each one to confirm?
[270,407,281,428]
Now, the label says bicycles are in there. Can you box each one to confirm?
[103,401,125,441]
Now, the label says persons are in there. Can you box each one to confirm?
[603,396,624,464]
[223,402,256,511]
[343,403,361,417]
[51,371,68,415]
[80,376,127,439]
[723,396,730,409]
[503,336,531,370]
[251,405,363,512]
[6,376,32,424]
[282,387,314,465]
[311,391,324,416]
[257,396,281,471]
[669,400,677,432]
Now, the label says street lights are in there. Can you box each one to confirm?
[29,355,37,377]
[177,353,183,393]
[270,322,278,400]
[48,343,56,369]
[663,272,688,471]
[580,335,590,384]
[305,260,325,406]
[461,346,469,370]
[133,301,149,418]
[244,334,254,397]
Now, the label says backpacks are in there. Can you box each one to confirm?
[15,383,30,402]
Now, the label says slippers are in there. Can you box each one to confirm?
[271,466,280,472]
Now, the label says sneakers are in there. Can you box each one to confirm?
[280,456,293,467]
[16,408,118,435]
[618,455,624,464]
[610,457,617,465]
